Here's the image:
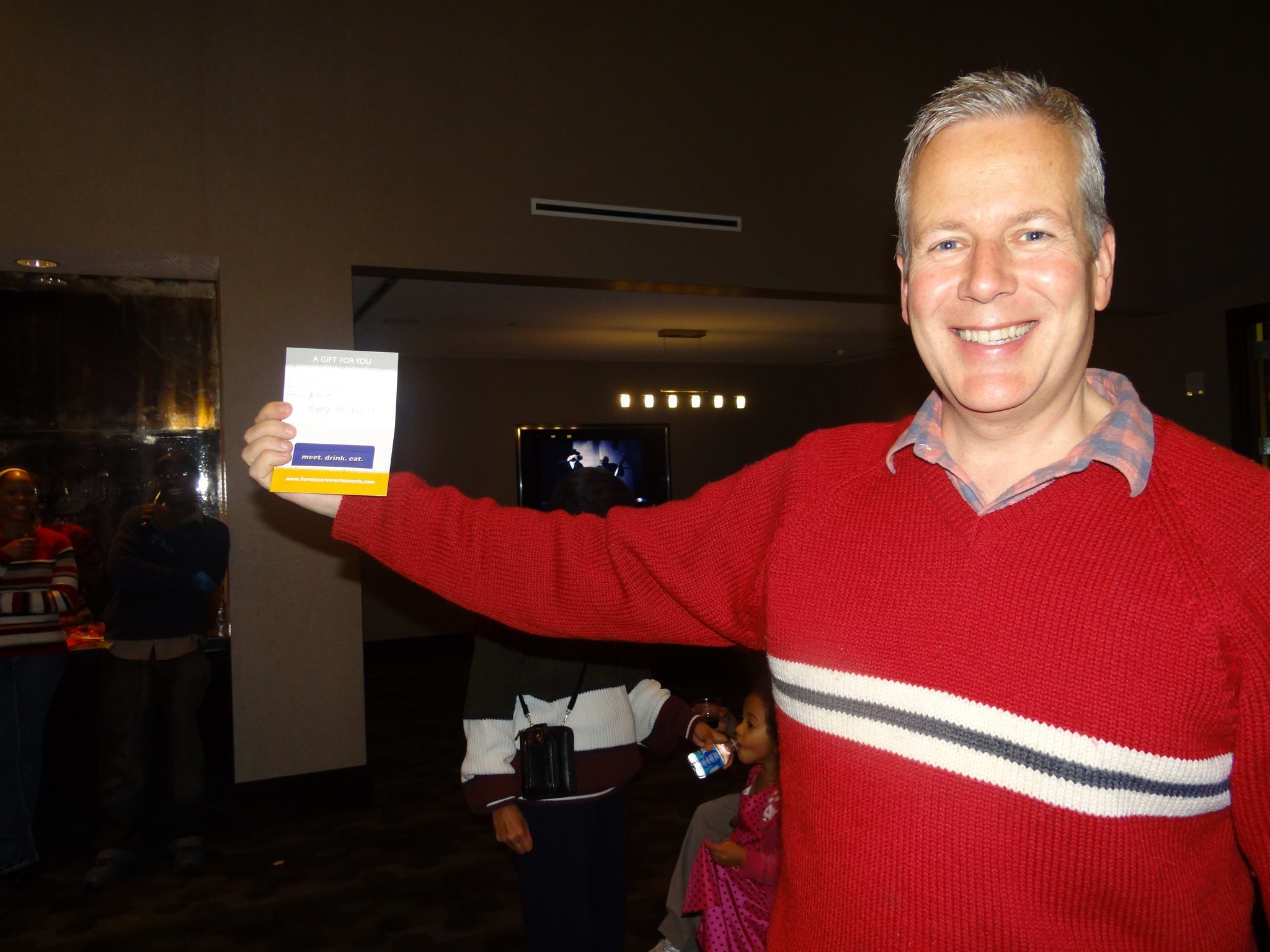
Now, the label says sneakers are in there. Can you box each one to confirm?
[169,836,207,875]
[82,848,136,889]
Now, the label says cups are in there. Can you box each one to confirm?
[691,698,721,729]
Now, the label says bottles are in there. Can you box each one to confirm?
[686,737,740,779]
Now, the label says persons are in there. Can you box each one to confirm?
[643,643,774,952]
[73,471,108,539]
[458,466,737,951]
[84,452,233,885]
[241,68,1270,952]
[38,472,107,628]
[593,455,625,477]
[679,683,783,952]
[0,463,79,897]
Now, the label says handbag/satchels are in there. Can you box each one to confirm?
[514,723,576,800]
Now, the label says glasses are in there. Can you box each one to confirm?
[155,468,191,483]
